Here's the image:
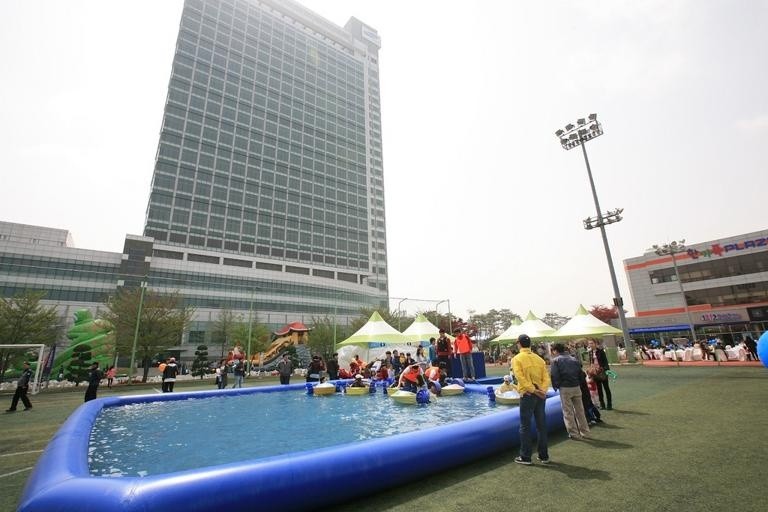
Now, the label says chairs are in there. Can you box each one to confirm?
[615,338,753,362]
[1,367,309,394]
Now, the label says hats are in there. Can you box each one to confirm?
[516,334,529,343]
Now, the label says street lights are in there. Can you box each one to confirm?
[554,112,637,364]
[652,238,699,344]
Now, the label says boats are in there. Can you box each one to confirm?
[494,387,520,404]
[342,381,377,395]
[427,378,464,395]
[390,387,437,403]
[309,381,341,394]
[383,381,399,397]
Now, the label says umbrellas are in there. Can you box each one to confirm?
[336,304,624,347]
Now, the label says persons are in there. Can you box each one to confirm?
[578,360,603,426]
[107,366,117,389]
[216,358,244,389]
[453,328,475,380]
[162,357,187,392]
[339,329,454,395]
[486,335,762,364]
[327,353,339,380]
[586,336,617,411]
[60,365,64,377]
[548,342,593,441]
[5,360,32,413]
[510,332,553,468]
[85,362,104,402]
[306,355,325,383]
[484,347,518,362]
[276,354,293,384]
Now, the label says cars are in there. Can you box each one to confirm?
[206,361,222,374]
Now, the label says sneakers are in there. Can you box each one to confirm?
[513,455,532,465]
[537,456,549,463]
